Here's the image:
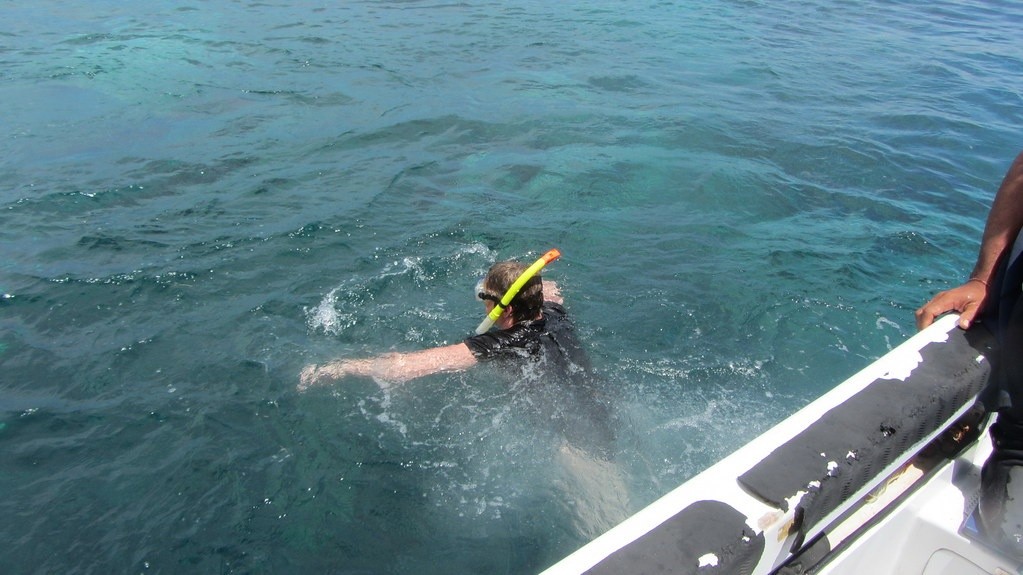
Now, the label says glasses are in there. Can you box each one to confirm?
[474,278,487,302]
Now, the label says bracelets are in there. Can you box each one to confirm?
[967,278,991,289]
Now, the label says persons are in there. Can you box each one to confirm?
[299,262,634,540]
[913,150,1023,557]
